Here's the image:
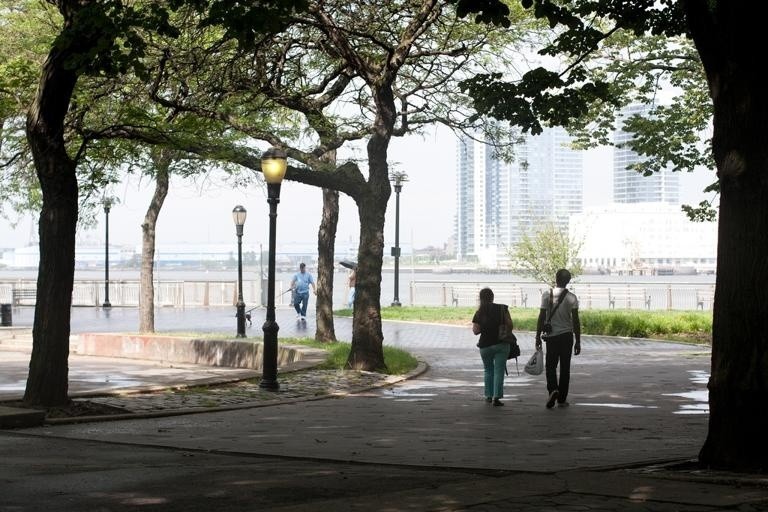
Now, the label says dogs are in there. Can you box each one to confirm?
[235,310,253,326]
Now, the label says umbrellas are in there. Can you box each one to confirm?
[339,259,358,271]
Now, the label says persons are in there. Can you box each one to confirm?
[347,268,357,313]
[290,262,317,322]
[534,269,582,410]
[471,287,513,407]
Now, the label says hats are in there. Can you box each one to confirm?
[300,263,305,267]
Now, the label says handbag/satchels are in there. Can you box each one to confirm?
[499,323,515,342]
[509,334,520,359]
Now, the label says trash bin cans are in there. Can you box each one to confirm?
[0,303,12,326]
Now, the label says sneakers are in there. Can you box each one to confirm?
[297,313,306,320]
[546,390,569,408]
[485,396,504,407]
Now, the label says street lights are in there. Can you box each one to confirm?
[390,171,404,306]
[231,204,247,340]
[100,197,114,309]
[258,146,290,390]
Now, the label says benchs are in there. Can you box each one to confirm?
[11,288,37,307]
